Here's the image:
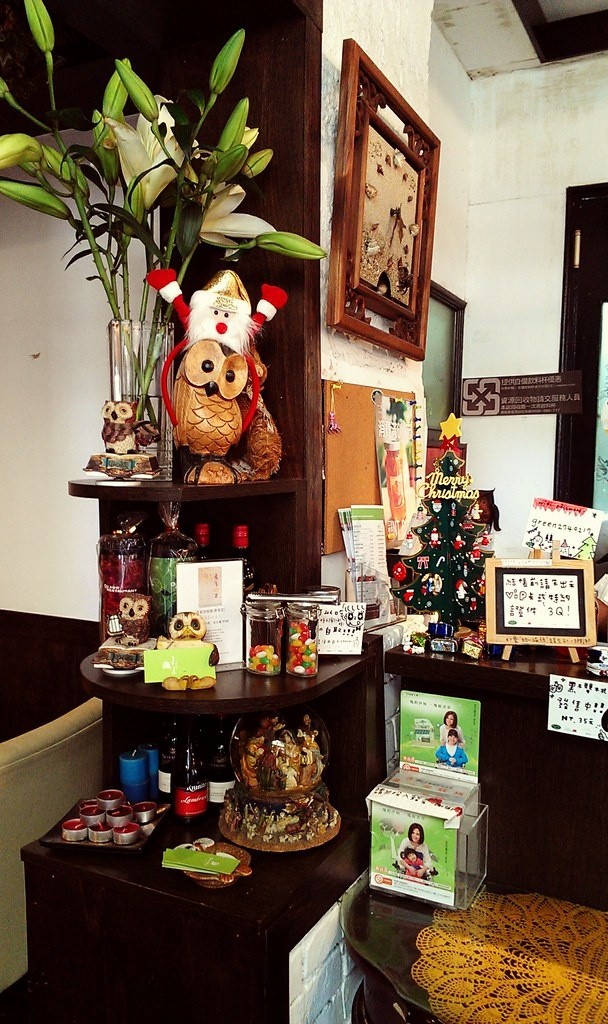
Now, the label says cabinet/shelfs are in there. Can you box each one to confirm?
[384,645,608,912]
[21,479,387,1024]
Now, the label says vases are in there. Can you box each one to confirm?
[108,319,175,481]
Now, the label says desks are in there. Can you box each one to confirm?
[344,880,608,1024]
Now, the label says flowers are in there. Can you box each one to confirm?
[0,0,327,322]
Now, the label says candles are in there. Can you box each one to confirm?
[79,805,105,825]
[121,779,149,802]
[119,749,148,786]
[62,818,88,842]
[133,801,158,823]
[138,743,158,776]
[77,799,97,811]
[148,776,160,799]
[87,820,114,842]
[105,805,134,827]
[112,822,141,846]
[96,789,124,811]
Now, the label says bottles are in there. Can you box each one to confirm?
[224,523,254,605]
[158,716,180,797]
[208,716,236,810]
[173,729,208,828]
[190,523,215,563]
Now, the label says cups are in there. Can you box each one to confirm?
[120,752,146,785]
[145,742,158,776]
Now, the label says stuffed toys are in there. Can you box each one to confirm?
[146,269,288,479]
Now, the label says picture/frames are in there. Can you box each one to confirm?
[325,40,441,361]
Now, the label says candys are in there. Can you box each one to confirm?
[247,620,316,676]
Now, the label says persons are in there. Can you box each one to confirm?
[436,711,468,768]
[246,713,324,789]
[398,823,433,885]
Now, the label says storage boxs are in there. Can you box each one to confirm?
[431,639,456,653]
[461,640,483,660]
[369,624,403,684]
[383,675,401,720]
[384,712,400,763]
[387,751,399,776]
[428,622,453,637]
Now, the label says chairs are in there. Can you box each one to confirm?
[0,696,102,995]
